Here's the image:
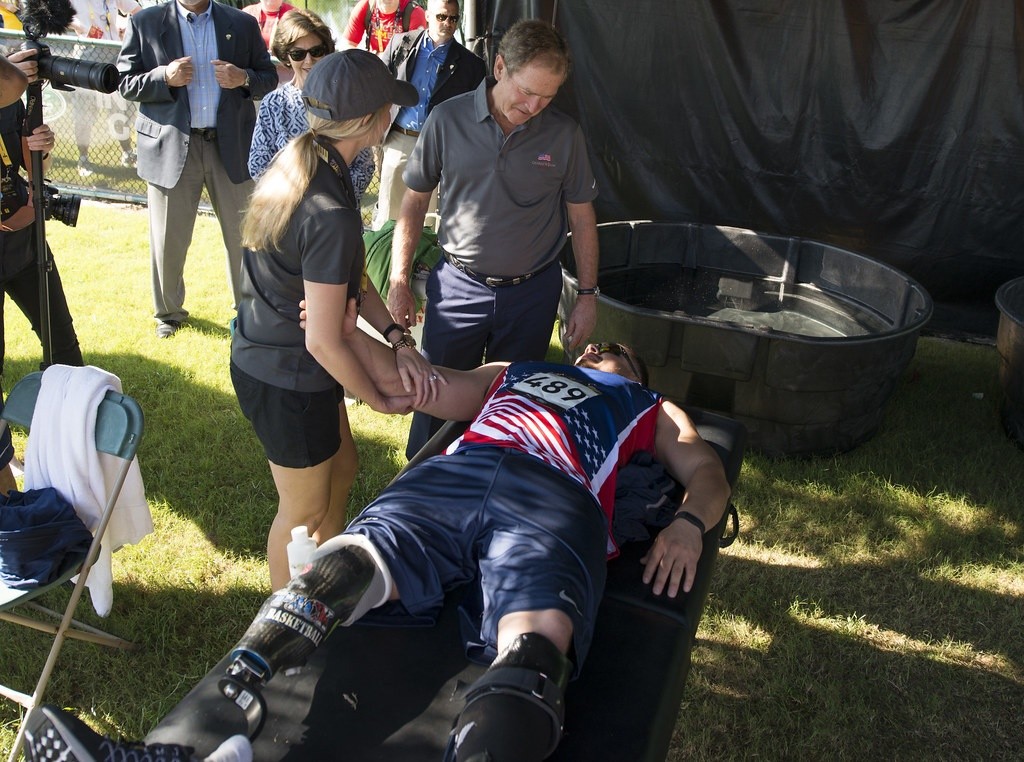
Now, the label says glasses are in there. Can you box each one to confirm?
[281,45,327,61]
[594,340,638,379]
[430,9,459,24]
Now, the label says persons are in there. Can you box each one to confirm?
[230,50,449,594]
[388,19,603,464]
[337,0,428,55]
[247,7,376,237]
[21,292,733,762]
[117,0,279,341]
[51,0,144,176]
[242,0,298,49]
[377,0,487,231]
[0,40,85,372]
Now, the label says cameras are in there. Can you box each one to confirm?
[20,40,120,94]
[42,185,82,228]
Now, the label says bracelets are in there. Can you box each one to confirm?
[383,323,405,342]
[674,511,707,536]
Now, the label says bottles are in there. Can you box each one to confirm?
[286,526,317,581]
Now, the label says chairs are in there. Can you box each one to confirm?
[0,370,144,762]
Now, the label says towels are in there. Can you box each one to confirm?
[19,362,156,620]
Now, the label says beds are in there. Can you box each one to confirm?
[144,403,749,762]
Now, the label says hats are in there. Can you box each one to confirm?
[301,49,420,120]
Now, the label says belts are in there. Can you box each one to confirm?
[442,250,554,288]
[392,124,421,138]
[190,127,218,141]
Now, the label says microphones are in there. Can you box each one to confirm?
[17,0,77,38]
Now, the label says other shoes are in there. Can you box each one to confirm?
[23,703,199,762]
[156,319,179,339]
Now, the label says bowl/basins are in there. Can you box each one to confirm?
[993,276,1024,448]
[557,220,933,456]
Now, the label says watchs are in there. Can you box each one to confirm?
[393,335,416,350]
[575,283,601,298]
[242,69,250,87]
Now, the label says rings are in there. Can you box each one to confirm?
[429,375,436,382]
[46,139,51,145]
[586,336,590,338]
